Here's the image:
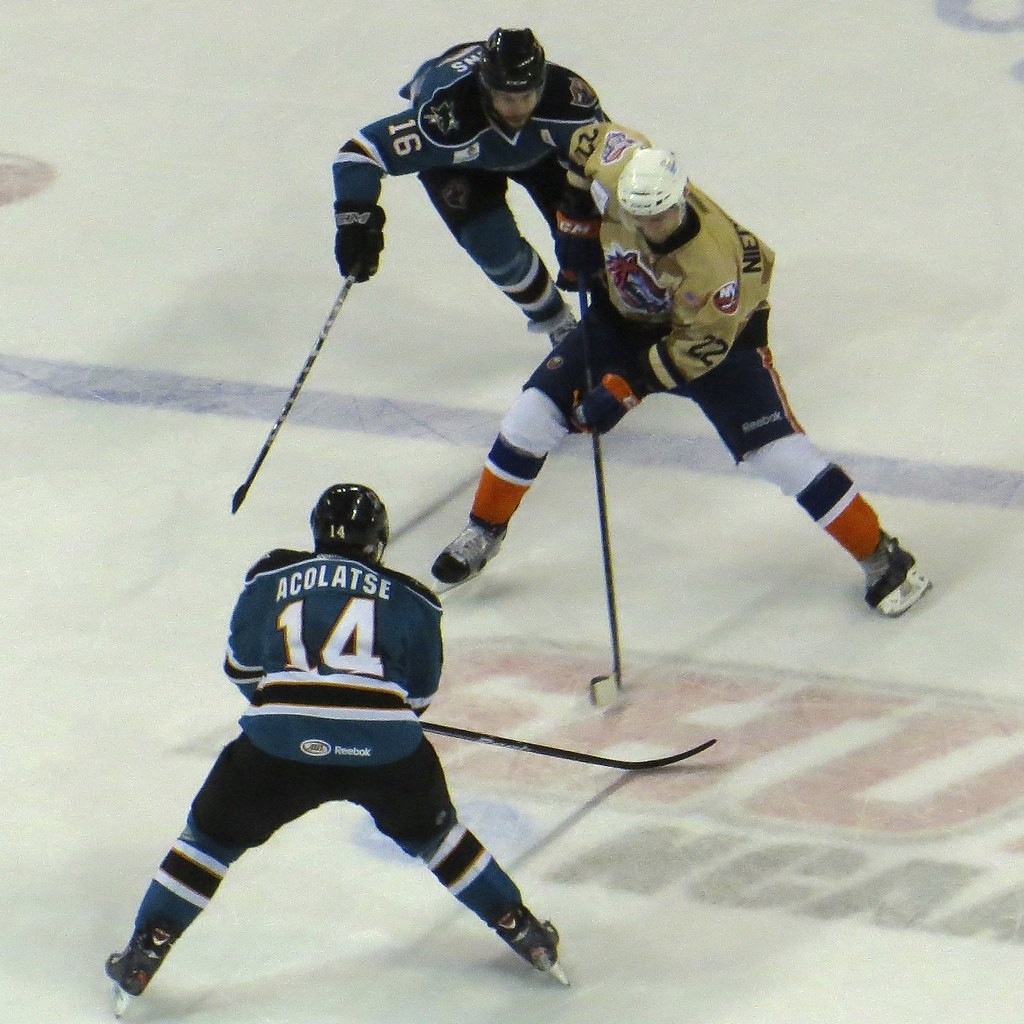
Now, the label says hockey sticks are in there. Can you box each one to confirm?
[230,235,376,516]
[573,267,625,708]
[416,722,718,772]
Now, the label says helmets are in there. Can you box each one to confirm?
[310,482,391,564]
[478,26,546,93]
[617,147,688,216]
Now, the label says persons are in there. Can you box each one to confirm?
[422,119,935,618]
[104,481,571,1023]
[333,27,617,345]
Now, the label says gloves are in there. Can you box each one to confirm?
[569,349,659,436]
[555,187,604,277]
[334,199,385,282]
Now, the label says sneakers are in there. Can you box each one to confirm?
[431,512,509,594]
[493,903,571,986]
[525,302,578,349]
[857,528,934,618]
[104,922,174,1019]
